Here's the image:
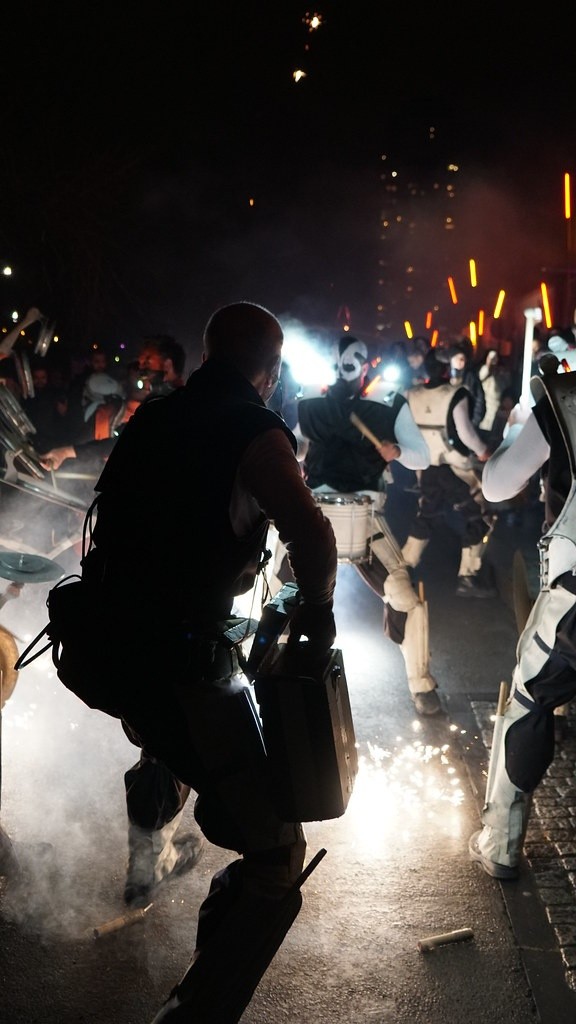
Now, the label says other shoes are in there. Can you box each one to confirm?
[468,830,521,880]
[414,689,440,717]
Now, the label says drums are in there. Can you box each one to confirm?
[0,308,123,481]
[309,492,371,563]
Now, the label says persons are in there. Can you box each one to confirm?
[0,301,575,1023]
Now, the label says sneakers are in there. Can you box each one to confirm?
[127,834,206,904]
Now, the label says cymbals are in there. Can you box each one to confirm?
[0,625,19,709]
[0,552,67,585]
